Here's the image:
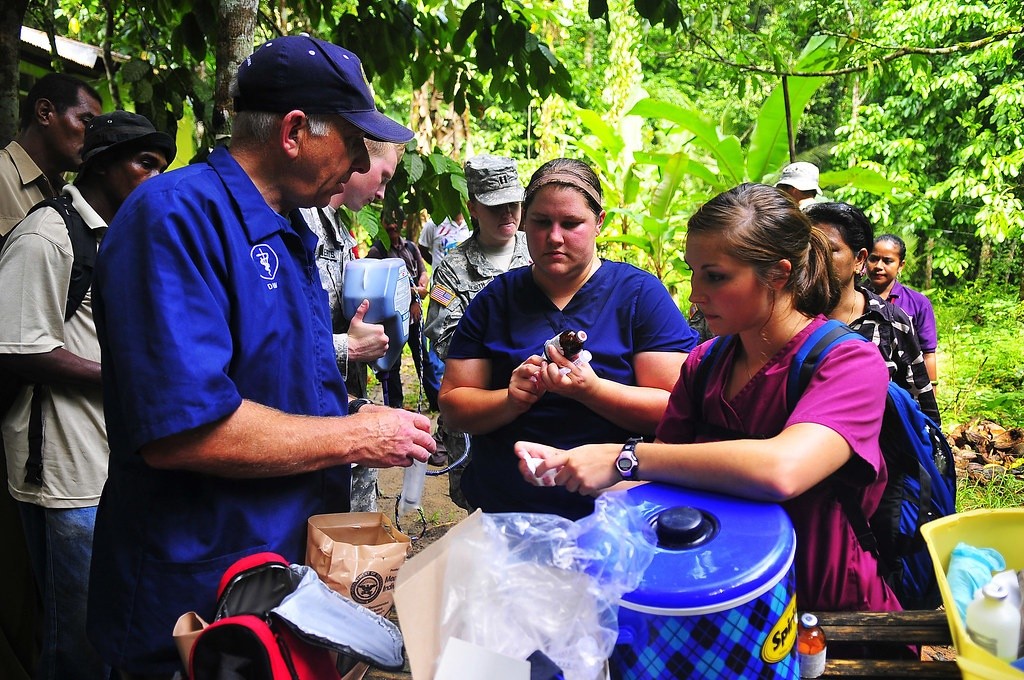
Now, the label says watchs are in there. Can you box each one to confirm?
[615,437,645,483]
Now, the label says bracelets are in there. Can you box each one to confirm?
[347,397,373,416]
[931,381,938,386]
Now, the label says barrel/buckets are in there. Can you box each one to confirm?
[577,482,800,680]
[344,258,410,380]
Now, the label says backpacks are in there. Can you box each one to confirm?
[689,324,956,611]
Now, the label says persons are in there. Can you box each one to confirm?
[437,160,698,525]
[0,110,178,680]
[514,162,940,661]
[85,34,436,680]
[0,72,104,249]
[298,133,531,512]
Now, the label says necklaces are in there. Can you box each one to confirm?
[742,315,802,380]
[847,290,856,324]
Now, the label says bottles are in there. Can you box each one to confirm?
[543,330,587,364]
[966,583,1020,663]
[800,613,827,679]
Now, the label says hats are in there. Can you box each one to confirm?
[82,110,178,168]
[774,162,823,196]
[234,36,415,141]
[466,155,526,205]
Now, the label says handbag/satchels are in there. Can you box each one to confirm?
[189,552,404,680]
